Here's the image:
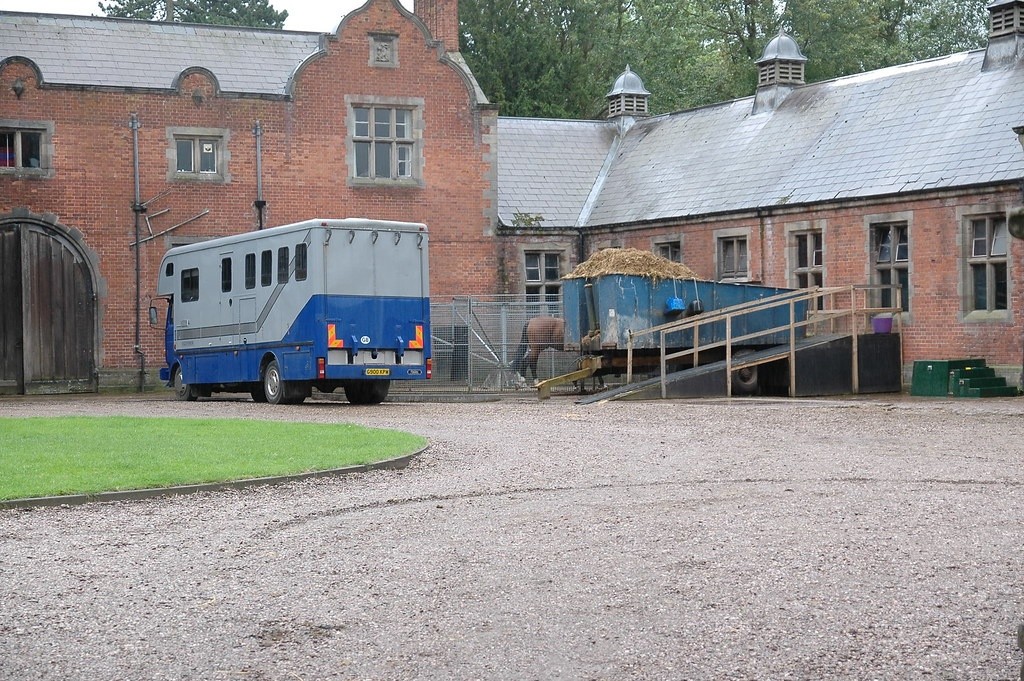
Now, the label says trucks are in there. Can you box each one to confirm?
[148,216,432,404]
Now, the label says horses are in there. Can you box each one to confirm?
[509,316,565,387]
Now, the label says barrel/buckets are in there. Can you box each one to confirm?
[871,318,893,333]
